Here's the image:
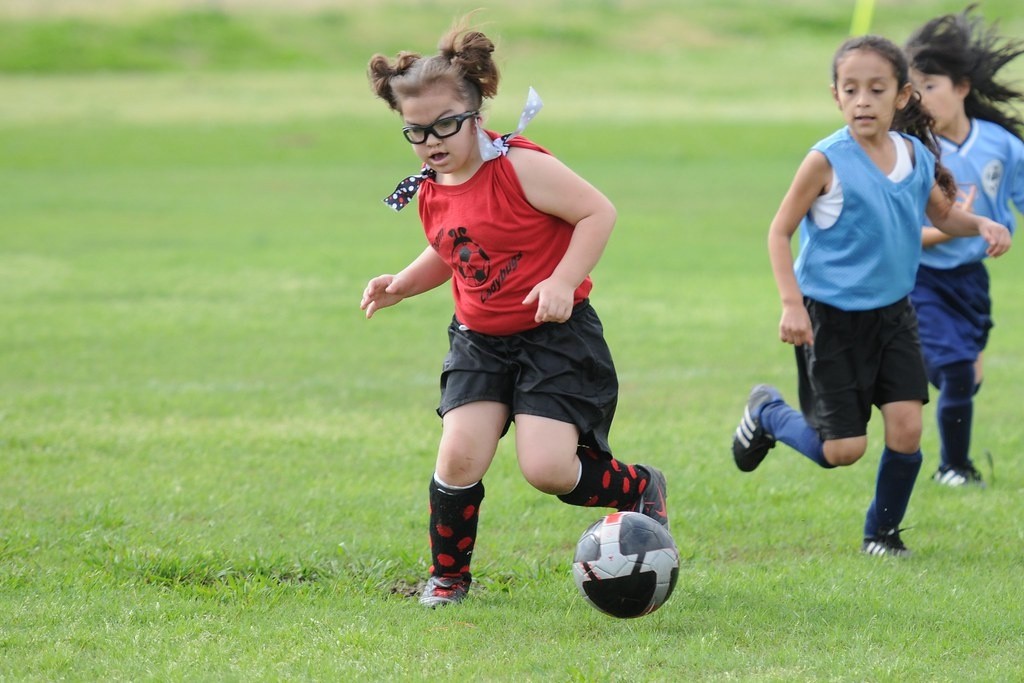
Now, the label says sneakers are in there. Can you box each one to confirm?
[617,464,668,533]
[862,527,912,558]
[419,576,472,609]
[733,384,784,472]
[933,462,986,492]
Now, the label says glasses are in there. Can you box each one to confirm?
[402,110,479,144]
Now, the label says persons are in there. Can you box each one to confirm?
[731,38,1011,558]
[900,4,1024,490]
[362,33,666,609]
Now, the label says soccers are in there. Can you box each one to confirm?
[570,509,684,621]
